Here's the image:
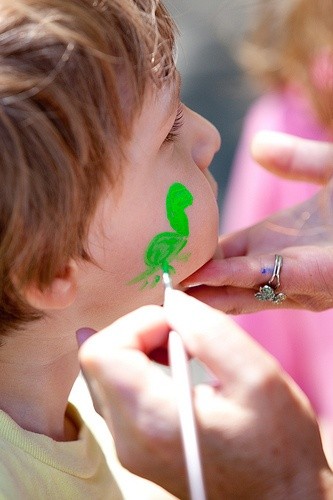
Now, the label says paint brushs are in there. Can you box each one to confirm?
[159,261,210,500]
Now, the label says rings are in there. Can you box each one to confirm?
[253,254,287,304]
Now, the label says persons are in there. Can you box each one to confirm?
[75,127,332,499]
[224,1,332,441]
[0,0,221,499]
